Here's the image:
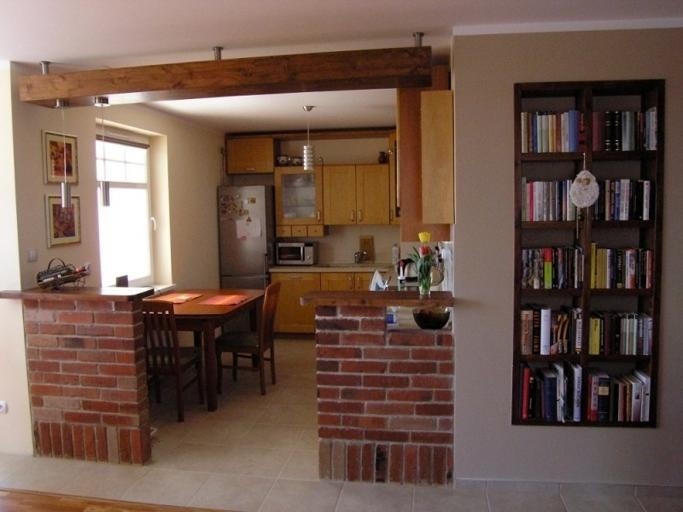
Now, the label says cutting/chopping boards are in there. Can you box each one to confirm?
[360,239,374,261]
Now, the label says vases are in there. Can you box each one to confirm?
[419,280,431,298]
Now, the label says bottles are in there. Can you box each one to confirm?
[37,264,89,289]
[392,244,399,264]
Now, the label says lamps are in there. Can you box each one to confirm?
[53,98,71,208]
[94,97,110,206]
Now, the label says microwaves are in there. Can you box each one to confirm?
[275,241,318,266]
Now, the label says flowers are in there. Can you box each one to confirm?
[407,231,437,296]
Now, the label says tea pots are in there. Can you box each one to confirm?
[378,151,388,164]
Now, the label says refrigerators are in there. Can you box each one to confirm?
[218,184,274,345]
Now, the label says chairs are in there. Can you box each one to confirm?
[116,275,281,422]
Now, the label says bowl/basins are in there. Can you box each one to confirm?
[412,309,450,329]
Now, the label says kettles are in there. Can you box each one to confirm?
[397,258,418,283]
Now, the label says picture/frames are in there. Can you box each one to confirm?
[41,129,78,185]
[46,194,81,248]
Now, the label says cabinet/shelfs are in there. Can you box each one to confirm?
[271,272,387,334]
[275,166,322,238]
[323,164,389,224]
[228,138,274,173]
[397,87,424,226]
